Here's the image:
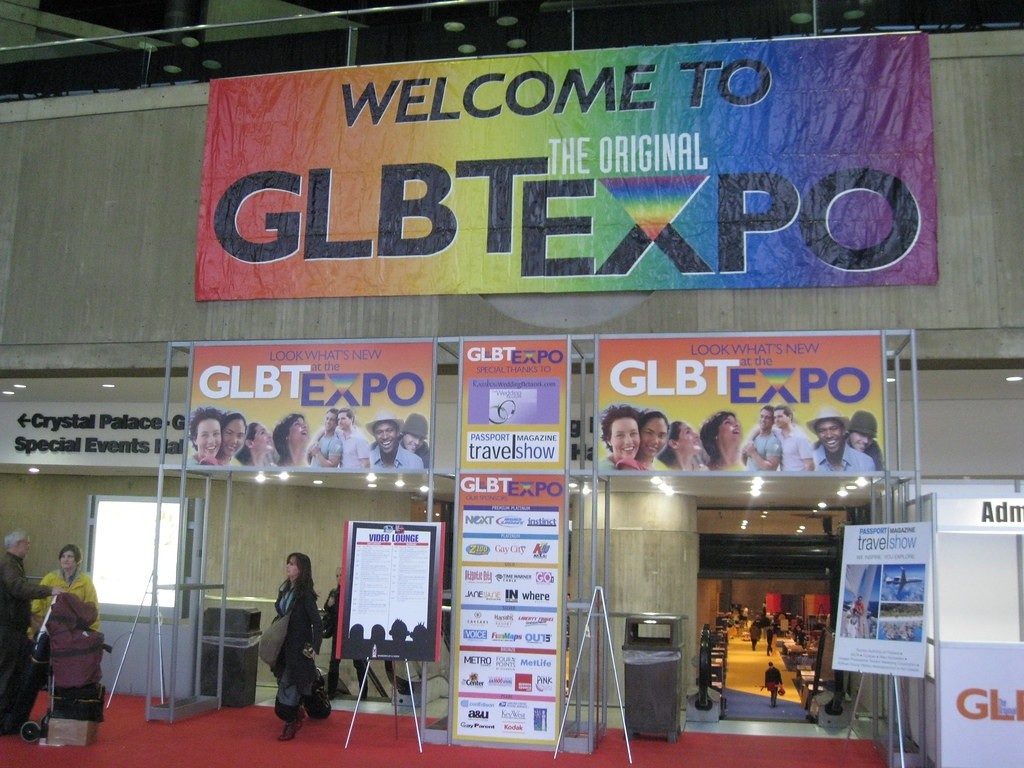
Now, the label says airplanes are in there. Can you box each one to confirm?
[885,566,923,593]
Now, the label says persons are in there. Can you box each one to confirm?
[0,528,68,724]
[188,403,431,473]
[599,399,885,473]
[849,593,865,621]
[724,599,806,657]
[324,566,369,703]
[764,660,783,708]
[30,543,101,647]
[266,552,323,740]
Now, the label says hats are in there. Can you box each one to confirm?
[402,414,428,439]
[410,622,427,637]
[389,619,410,636]
[806,406,849,435]
[366,410,404,438]
[848,410,878,438]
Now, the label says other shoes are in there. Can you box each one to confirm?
[279,718,302,740]
[767,653,770,656]
[769,702,772,707]
[296,708,306,730]
[771,648,773,653]
[773,701,775,707]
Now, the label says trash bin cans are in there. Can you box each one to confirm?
[200,606,262,708]
[621,613,688,744]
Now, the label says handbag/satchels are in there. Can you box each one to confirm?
[322,614,337,638]
[50,684,106,723]
[259,613,290,666]
[767,682,776,691]
[778,687,785,695]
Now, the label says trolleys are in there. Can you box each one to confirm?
[19,595,57,744]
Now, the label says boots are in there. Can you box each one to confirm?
[357,672,368,701]
[328,672,339,700]
